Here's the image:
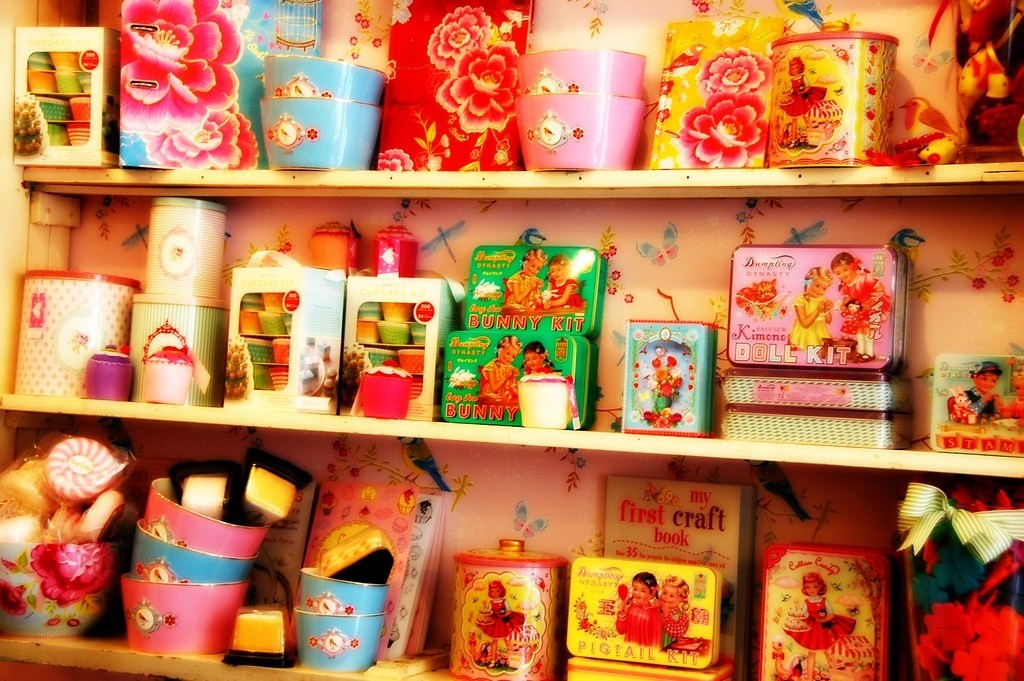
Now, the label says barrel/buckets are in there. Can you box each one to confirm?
[771,22,898,167]
[450,539,566,681]
[14,269,142,398]
[130,295,231,408]
[144,199,227,300]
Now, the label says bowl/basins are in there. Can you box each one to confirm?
[265,55,386,106]
[517,49,647,100]
[121,573,251,655]
[294,606,384,673]
[260,97,382,171]
[0,540,119,638]
[300,568,390,614]
[516,93,645,171]
[143,478,272,557]
[131,518,260,583]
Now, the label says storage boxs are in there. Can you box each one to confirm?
[225,242,1023,458]
[563,471,1024,681]
[15,25,120,167]
[120,1,321,169]
[651,15,900,175]
[374,0,540,171]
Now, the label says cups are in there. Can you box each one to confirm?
[373,225,418,278]
[360,361,411,419]
[309,221,355,276]
[143,346,193,406]
[518,373,571,429]
[87,346,131,402]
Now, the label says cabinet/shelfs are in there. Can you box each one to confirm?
[0,0,1024,681]
